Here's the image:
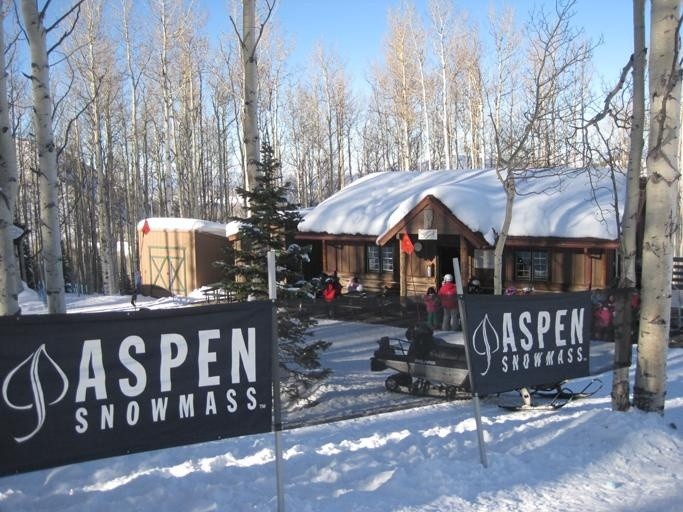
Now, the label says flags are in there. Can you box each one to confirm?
[400,229,414,255]
[142,219,151,234]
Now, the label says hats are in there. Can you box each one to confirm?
[444,274,453,281]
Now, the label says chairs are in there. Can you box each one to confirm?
[325,290,392,320]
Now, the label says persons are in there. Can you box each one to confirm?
[322,285,336,319]
[345,276,362,293]
[591,287,639,342]
[422,287,439,330]
[129,269,141,307]
[437,275,457,330]
